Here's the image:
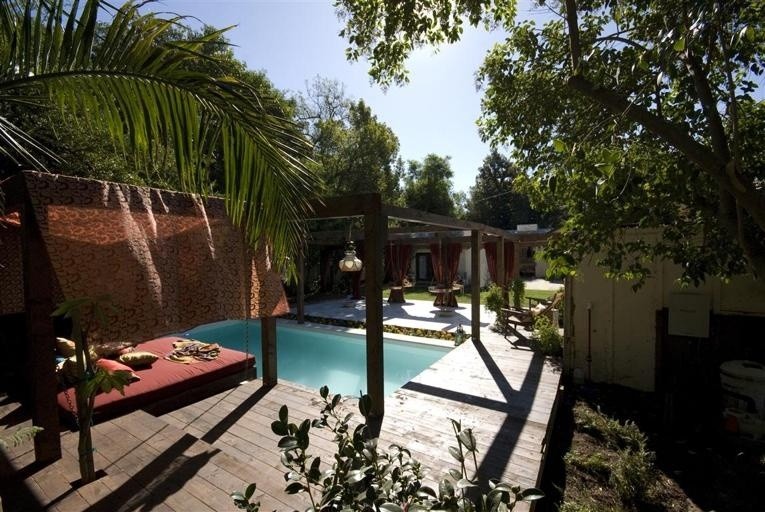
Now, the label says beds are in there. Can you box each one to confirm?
[57,336,259,433]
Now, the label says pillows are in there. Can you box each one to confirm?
[92,339,135,358]
[96,358,134,375]
[55,336,75,356]
[117,350,160,366]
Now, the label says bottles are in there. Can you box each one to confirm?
[457,324,464,334]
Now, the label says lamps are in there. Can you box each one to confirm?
[337,240,363,281]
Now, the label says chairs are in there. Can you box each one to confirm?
[499,288,565,352]
